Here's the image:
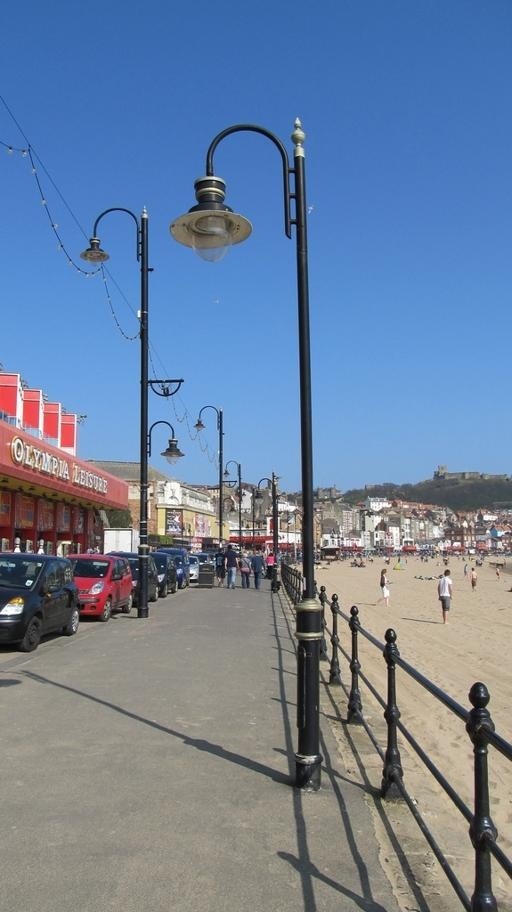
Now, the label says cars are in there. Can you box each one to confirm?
[0,541,218,647]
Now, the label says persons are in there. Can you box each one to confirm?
[214,534,512,609]
[90,546,99,554]
[439,567,454,625]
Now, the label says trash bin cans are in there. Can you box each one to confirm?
[199,563,216,588]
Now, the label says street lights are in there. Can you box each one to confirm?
[193,405,238,540]
[254,474,280,593]
[78,206,185,619]
[164,114,323,793]
[224,458,250,551]
